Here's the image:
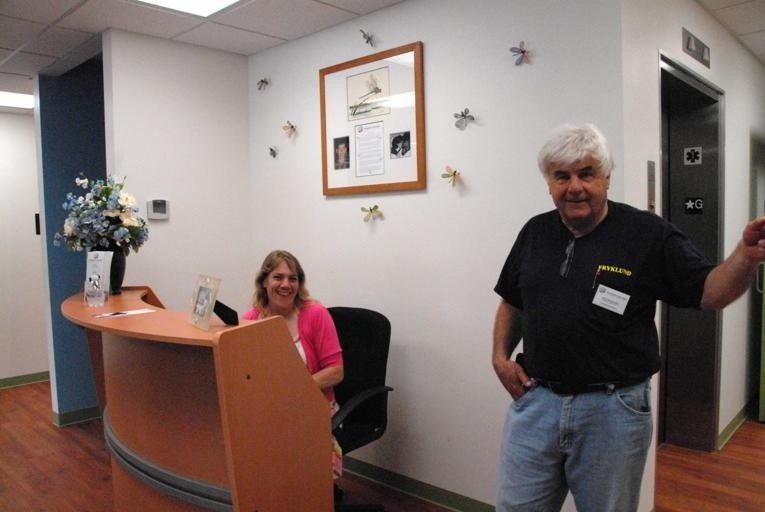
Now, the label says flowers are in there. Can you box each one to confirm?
[52,170,149,257]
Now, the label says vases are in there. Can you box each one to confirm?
[92,243,126,295]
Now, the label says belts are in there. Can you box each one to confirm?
[538,380,625,394]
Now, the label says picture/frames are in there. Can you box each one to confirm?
[187,272,221,332]
[320,41,425,195]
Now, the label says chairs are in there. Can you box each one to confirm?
[320,307,394,512]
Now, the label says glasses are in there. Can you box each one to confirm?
[559,237,576,278]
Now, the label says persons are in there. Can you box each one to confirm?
[492,124,765,511]
[335,140,349,170]
[241,251,344,505]
